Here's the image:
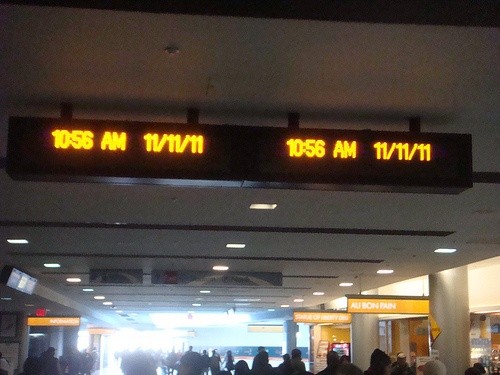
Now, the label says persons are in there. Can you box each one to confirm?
[0,346,488,375]
